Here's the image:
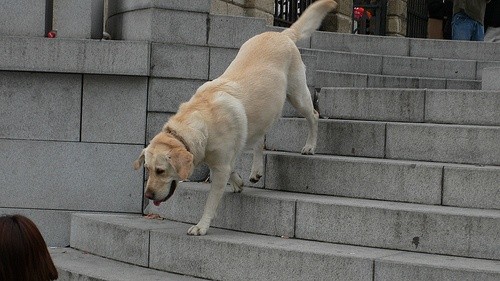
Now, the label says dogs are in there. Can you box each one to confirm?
[133,1,338,236]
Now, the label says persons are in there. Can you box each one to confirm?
[428,0,500,42]
[0,214,58,281]
